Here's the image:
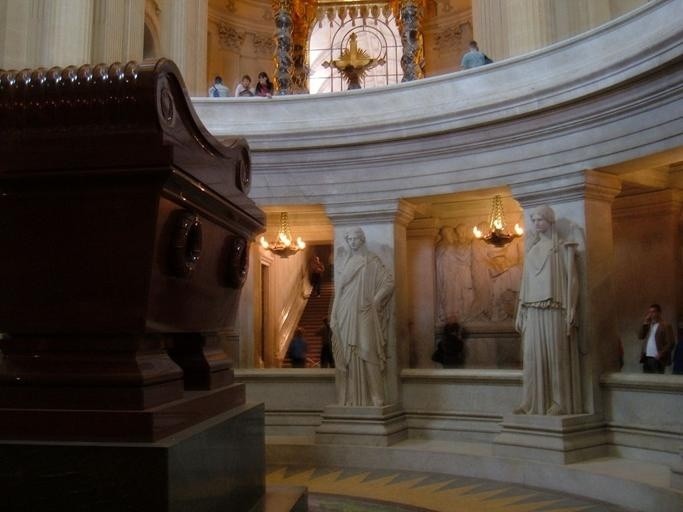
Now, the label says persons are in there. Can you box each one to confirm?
[635,303,676,374]
[512,204,583,414]
[308,256,325,299]
[432,220,513,324]
[287,326,308,368]
[431,320,465,368]
[314,315,335,368]
[254,71,274,98]
[207,76,231,97]
[457,40,493,71]
[328,226,396,408]
[408,321,425,368]
[234,74,255,96]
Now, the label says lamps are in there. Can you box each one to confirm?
[260,212,306,258]
[472,195,524,248]
